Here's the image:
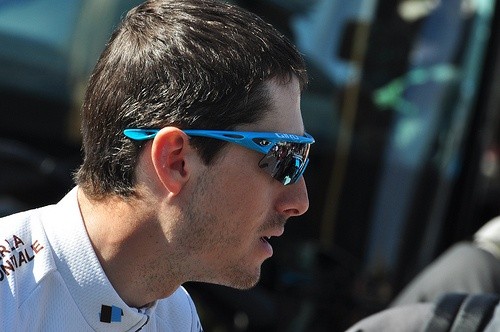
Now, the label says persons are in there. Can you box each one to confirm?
[2,0,312,332]
[340,217,500,332]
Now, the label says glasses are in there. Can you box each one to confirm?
[123,128,316,185]
